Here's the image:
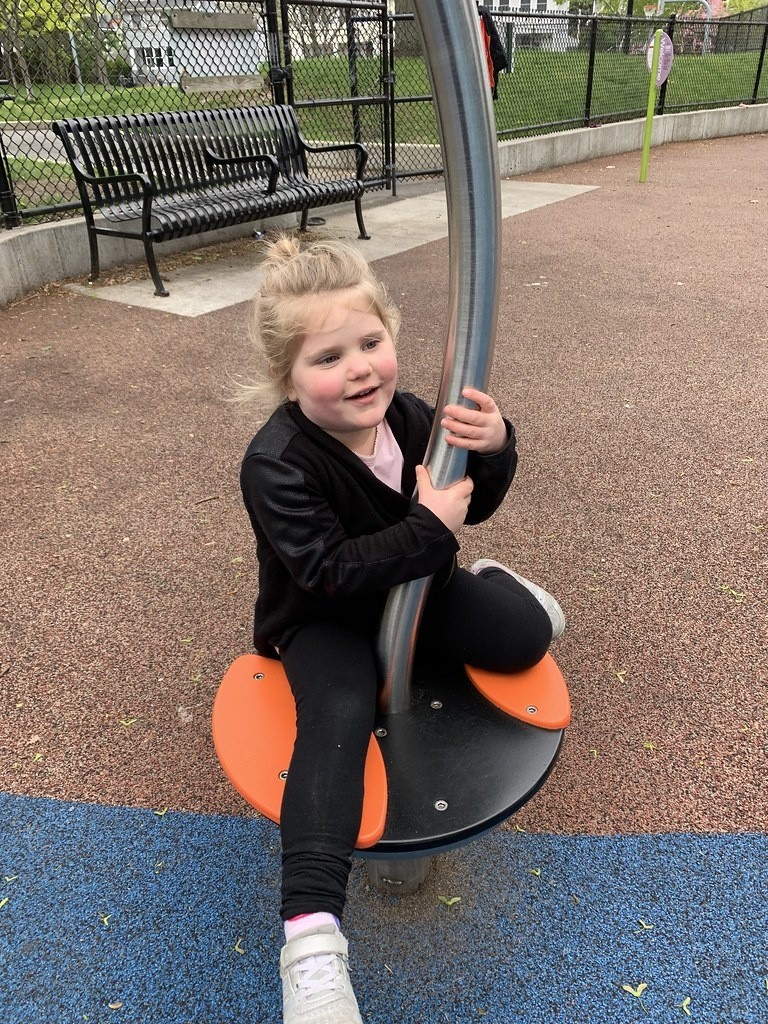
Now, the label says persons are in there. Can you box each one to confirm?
[239,240,566,1024]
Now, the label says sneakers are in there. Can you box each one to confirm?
[279,913,362,1024]
[470,558,565,640]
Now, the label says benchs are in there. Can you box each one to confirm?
[52,104,370,298]
